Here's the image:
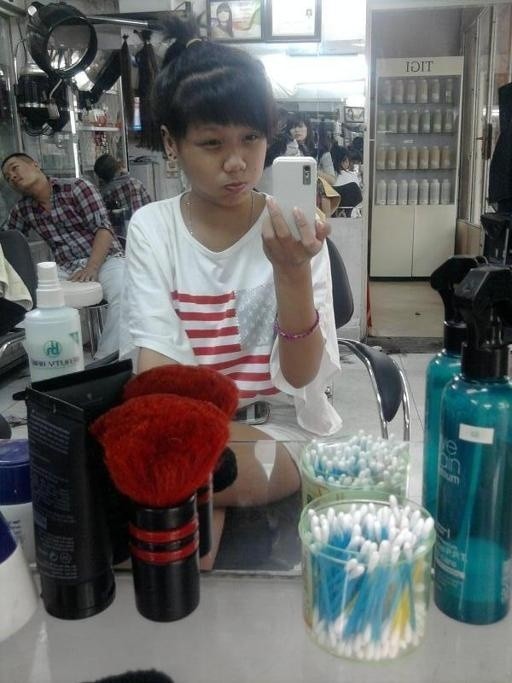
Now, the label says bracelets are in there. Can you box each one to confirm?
[272,307,321,340]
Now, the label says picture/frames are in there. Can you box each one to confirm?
[344,106,364,123]
[207,0,321,44]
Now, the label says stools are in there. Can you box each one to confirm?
[78,298,109,360]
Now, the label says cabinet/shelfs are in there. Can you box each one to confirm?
[129,164,160,202]
[11,75,128,178]
[275,98,345,149]
[370,56,464,278]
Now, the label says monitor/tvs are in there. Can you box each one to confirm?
[272,156,318,242]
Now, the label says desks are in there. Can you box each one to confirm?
[1,424,512,683]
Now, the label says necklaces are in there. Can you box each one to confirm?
[186,191,257,238]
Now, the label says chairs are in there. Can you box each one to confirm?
[84,236,409,441]
[1,230,38,427]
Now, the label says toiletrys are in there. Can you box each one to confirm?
[433,264,511,625]
[420,255,490,531]
[30,387,116,619]
[376,78,457,205]
[0,438,35,568]
[31,358,134,566]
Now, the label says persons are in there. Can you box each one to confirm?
[1,244,37,439]
[217,3,233,36]
[120,12,339,573]
[286,113,337,184]
[331,143,363,189]
[88,154,154,244]
[1,152,124,360]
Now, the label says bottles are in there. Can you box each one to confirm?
[380,77,452,103]
[202,465,216,558]
[376,179,451,204]
[1,511,38,646]
[22,259,86,380]
[377,108,453,130]
[1,437,42,566]
[377,145,452,168]
[128,491,200,625]
